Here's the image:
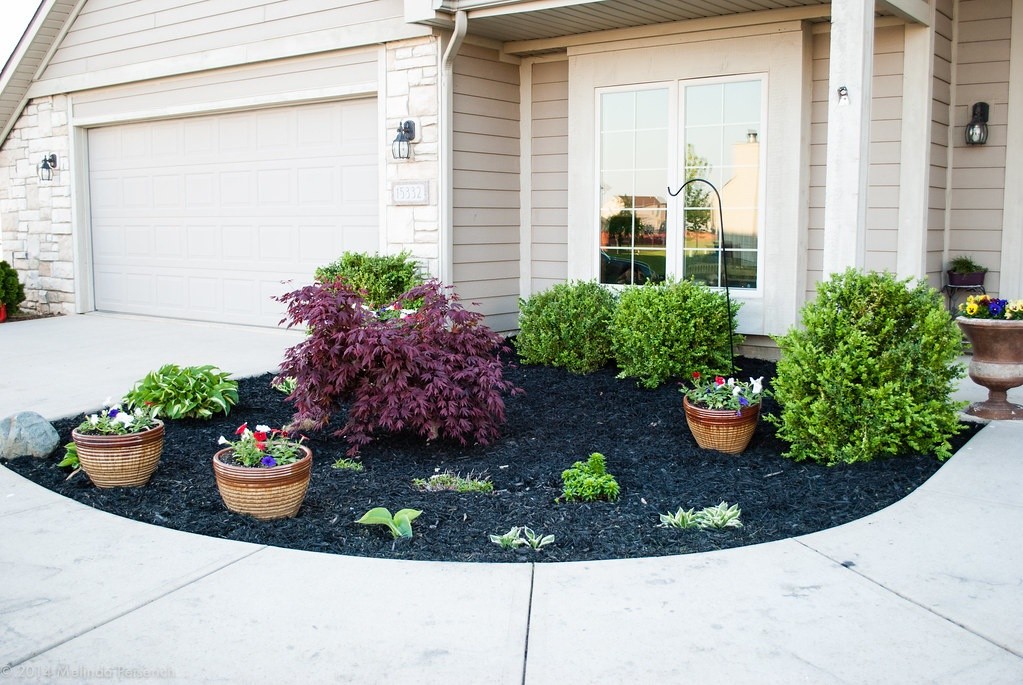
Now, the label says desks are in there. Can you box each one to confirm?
[944,285,985,309]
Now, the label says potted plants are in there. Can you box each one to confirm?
[949,257,988,285]
[0,260,26,322]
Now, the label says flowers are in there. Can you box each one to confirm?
[677,372,763,417]
[78,395,161,436]
[958,295,1023,320]
[218,423,310,468]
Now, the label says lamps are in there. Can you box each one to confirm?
[39,154,56,179]
[965,102,989,146]
[392,120,415,159]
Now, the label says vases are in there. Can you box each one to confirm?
[72,419,166,489]
[213,442,312,520]
[683,395,760,454]
[957,316,1023,420]
